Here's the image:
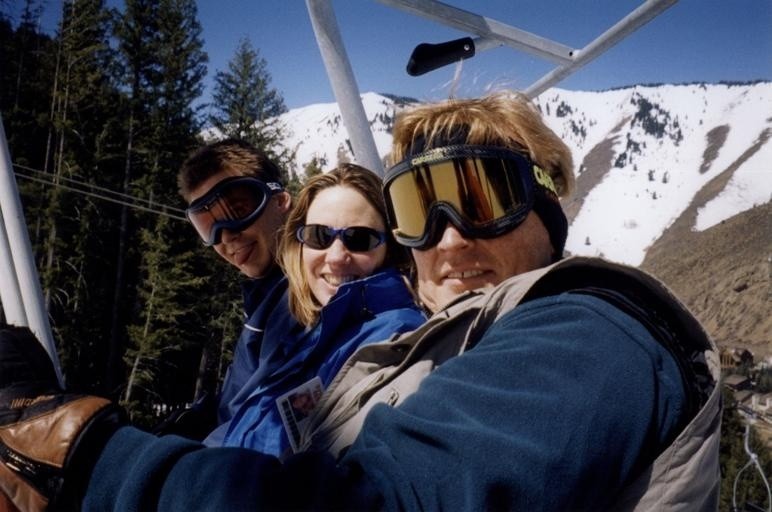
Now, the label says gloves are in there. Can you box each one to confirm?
[0,325,130,511]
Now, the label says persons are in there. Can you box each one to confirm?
[0,54,725,512]
[291,393,310,416]
[164,136,297,442]
[201,163,430,465]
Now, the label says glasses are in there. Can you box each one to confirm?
[381,144,558,251]
[185,176,285,248]
[296,224,386,252]
